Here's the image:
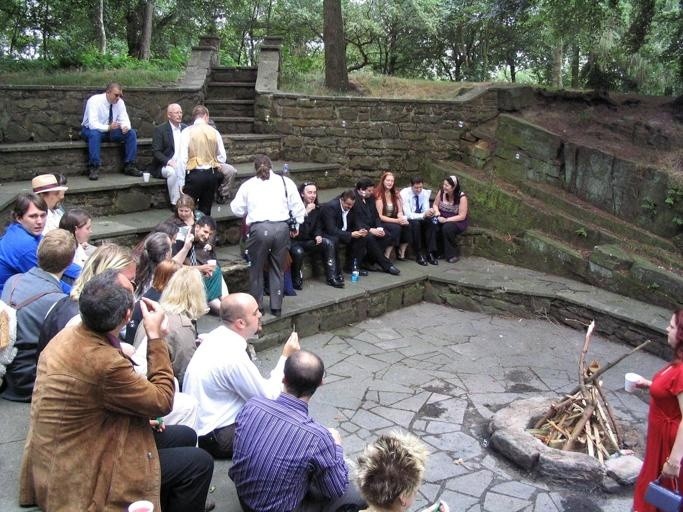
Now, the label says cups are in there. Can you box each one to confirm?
[207,259,216,266]
[142,172,150,182]
[624,372,642,393]
[128,500,155,512]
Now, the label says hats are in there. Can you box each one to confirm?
[31,174,69,193]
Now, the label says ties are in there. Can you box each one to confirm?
[414,195,421,213]
[109,103,114,125]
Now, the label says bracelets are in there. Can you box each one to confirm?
[665,457,680,469]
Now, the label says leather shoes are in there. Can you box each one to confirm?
[376,253,400,275]
[270,308,282,317]
[208,303,222,316]
[327,274,346,288]
[397,255,409,261]
[448,255,459,263]
[292,278,303,290]
[259,308,265,315]
[416,252,446,266]
[343,265,368,276]
[359,262,377,271]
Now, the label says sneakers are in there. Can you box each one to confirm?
[205,493,216,511]
[122,162,143,177]
[89,167,99,180]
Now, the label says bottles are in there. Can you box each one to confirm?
[351,258,359,283]
[282,163,289,174]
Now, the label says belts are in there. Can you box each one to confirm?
[186,168,216,173]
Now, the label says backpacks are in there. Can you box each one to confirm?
[0,274,65,388]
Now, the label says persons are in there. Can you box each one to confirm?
[632,308,683,512]
